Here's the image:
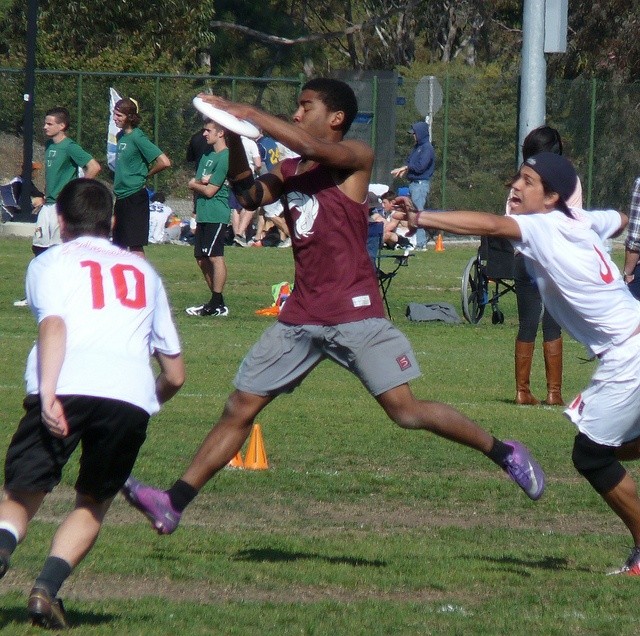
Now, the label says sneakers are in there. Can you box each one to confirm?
[233,234,249,248]
[184,303,221,317]
[604,535,640,576]
[232,242,241,248]
[28,587,67,631]
[121,474,181,535]
[199,305,229,316]
[280,237,293,248]
[500,440,545,501]
[277,241,284,248]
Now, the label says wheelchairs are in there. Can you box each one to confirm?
[461,234,519,326]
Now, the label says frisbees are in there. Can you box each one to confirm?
[192,96,259,139]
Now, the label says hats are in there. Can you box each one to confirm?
[524,151,577,219]
[114,97,143,122]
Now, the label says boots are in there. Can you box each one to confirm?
[542,335,566,406]
[514,333,540,405]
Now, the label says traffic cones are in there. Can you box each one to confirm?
[435,233,445,252]
[243,423,268,469]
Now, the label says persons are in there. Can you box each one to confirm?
[618,164,640,302]
[228,131,261,247]
[249,132,293,247]
[113,98,171,254]
[125,77,548,537]
[0,178,184,628]
[385,152,640,578]
[505,124,581,406]
[148,192,182,244]
[13,106,101,308]
[391,121,435,252]
[186,118,233,317]
[376,189,420,251]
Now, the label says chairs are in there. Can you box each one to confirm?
[368,221,415,323]
